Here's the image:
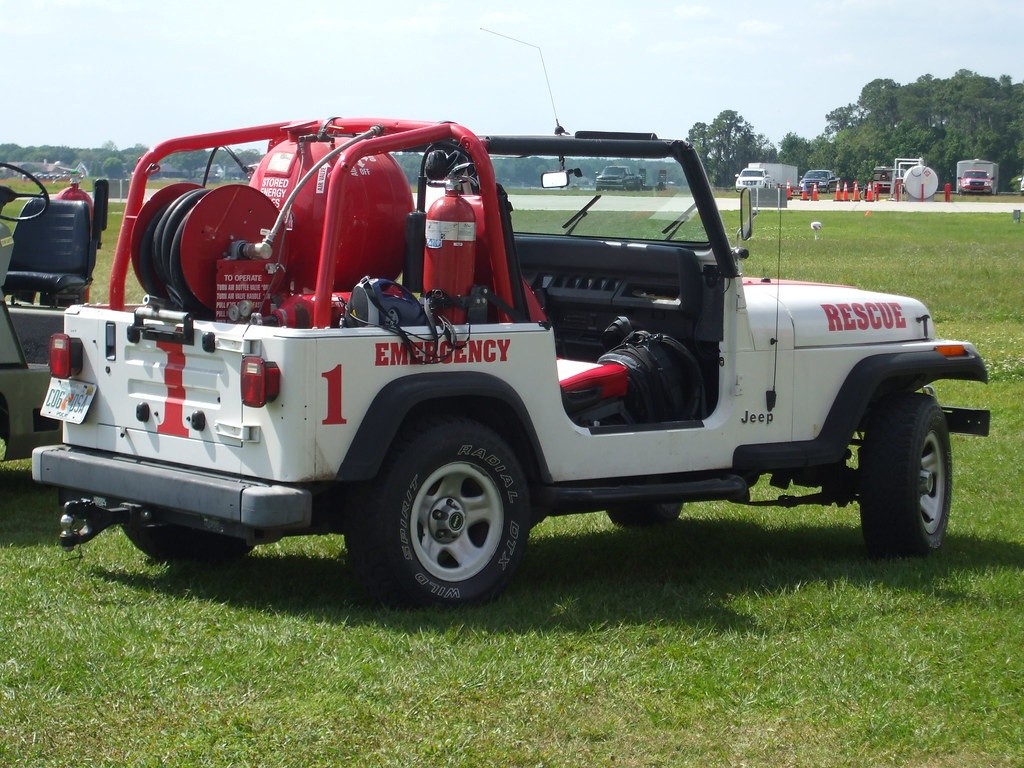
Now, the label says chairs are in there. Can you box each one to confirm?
[463,193,633,424]
[2,199,92,295]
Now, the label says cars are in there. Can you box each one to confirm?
[956,170,996,195]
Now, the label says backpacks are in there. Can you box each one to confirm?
[596,330,708,420]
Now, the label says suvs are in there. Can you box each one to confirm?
[32,115,994,610]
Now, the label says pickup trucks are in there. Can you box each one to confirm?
[594,165,645,191]
[799,165,841,195]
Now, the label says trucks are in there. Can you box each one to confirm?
[733,159,798,195]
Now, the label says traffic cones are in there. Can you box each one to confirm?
[853,183,861,201]
[800,179,809,201]
[812,183,819,201]
[834,180,841,201]
[785,178,794,200]
[865,182,873,202]
[843,181,850,201]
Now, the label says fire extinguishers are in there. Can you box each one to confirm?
[422,162,479,324]
